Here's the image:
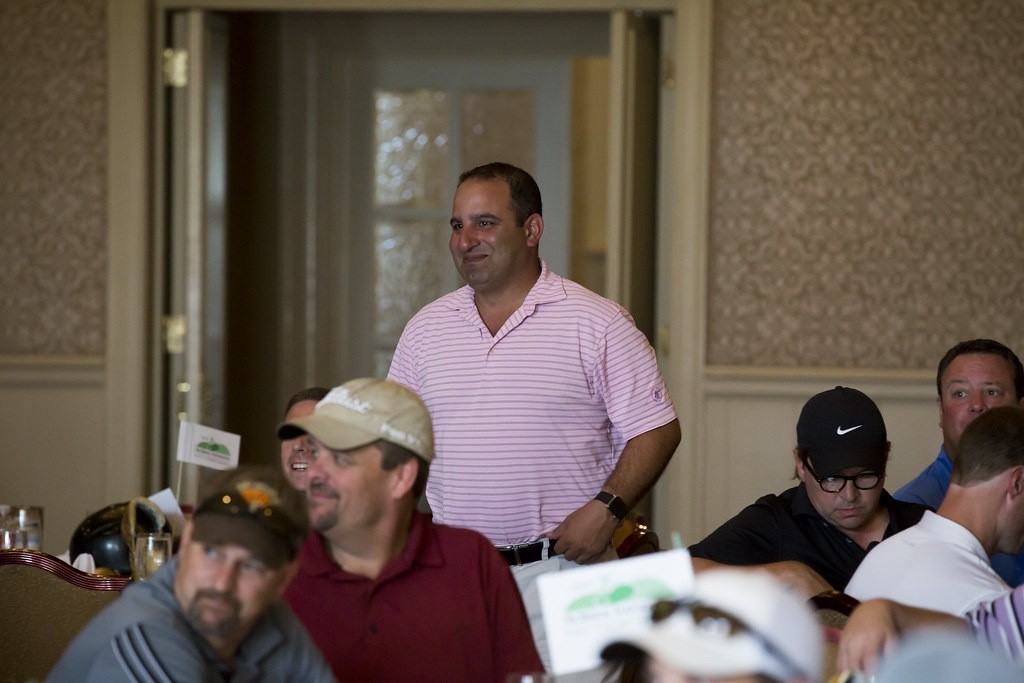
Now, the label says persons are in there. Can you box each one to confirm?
[386,162,681,683]
[279,377,547,683]
[839,407,1024,683]
[686,386,936,595]
[892,339,1024,589]
[43,466,337,683]
[233,387,334,530]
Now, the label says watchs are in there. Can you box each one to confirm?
[594,491,627,522]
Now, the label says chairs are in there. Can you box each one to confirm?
[0,548,135,683]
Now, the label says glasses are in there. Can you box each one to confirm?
[649,600,806,679]
[191,491,299,564]
[800,454,886,493]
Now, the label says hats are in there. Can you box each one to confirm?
[598,568,825,683]
[796,385,888,476]
[277,376,435,463]
[189,467,308,564]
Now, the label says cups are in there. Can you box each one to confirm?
[0,505,43,551]
[135,533,171,580]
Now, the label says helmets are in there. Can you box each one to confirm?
[69,498,174,582]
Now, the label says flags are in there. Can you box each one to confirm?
[176,421,240,471]
[538,548,697,675]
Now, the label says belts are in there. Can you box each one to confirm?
[497,538,562,566]
